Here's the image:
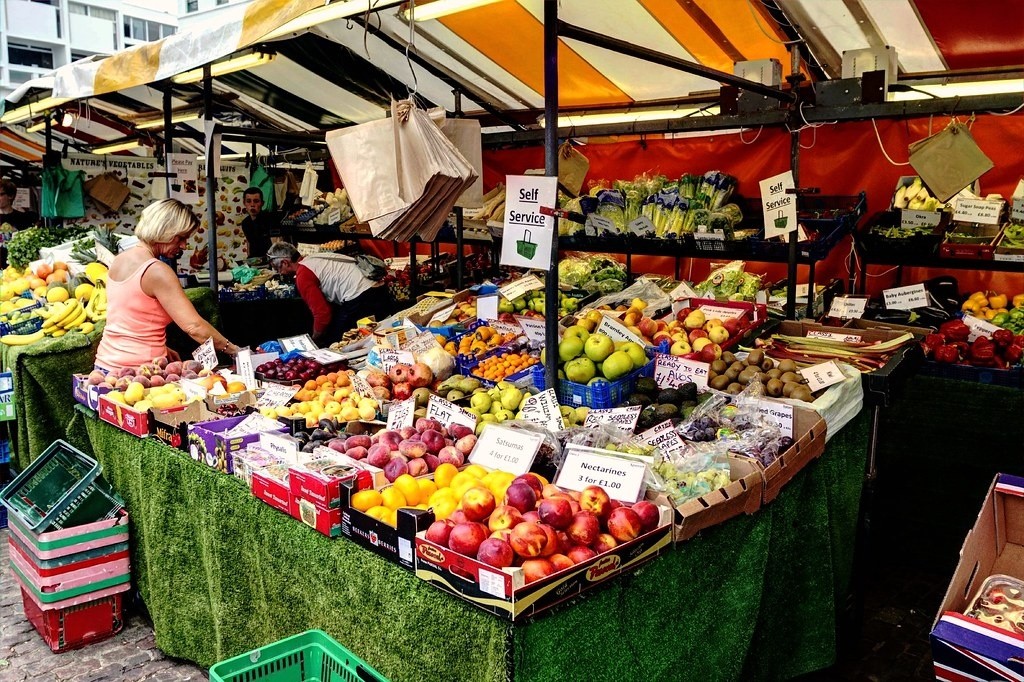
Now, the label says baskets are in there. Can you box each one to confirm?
[209,628,392,682]
[0,439,134,656]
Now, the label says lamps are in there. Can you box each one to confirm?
[170,45,278,85]
[87,136,152,155]
[397,0,502,26]
[134,107,203,130]
[536,105,720,129]
[25,113,73,135]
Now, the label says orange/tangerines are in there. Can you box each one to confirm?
[471,352,542,384]
[292,368,356,402]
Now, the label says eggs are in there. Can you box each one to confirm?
[319,240,354,252]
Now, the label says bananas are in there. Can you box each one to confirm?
[0,279,109,346]
[892,174,972,216]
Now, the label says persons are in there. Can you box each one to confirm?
[241,187,290,263]
[0,178,34,271]
[92,195,253,378]
[266,240,398,348]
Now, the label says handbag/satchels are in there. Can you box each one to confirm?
[355,253,388,282]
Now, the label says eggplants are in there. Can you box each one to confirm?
[864,276,969,331]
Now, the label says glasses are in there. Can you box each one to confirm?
[265,253,293,262]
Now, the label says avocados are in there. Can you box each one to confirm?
[618,377,712,435]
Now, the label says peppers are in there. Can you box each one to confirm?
[911,289,1024,371]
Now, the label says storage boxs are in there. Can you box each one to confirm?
[1,172,1024,681]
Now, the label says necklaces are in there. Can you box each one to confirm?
[135,243,155,258]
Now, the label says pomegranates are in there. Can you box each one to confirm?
[365,363,444,404]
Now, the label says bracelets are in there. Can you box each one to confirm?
[220,339,230,353]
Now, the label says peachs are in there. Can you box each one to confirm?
[90,357,213,394]
[258,389,660,585]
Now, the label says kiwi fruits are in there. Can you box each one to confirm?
[704,349,829,403]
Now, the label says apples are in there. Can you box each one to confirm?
[255,357,346,382]
[540,299,750,407]
